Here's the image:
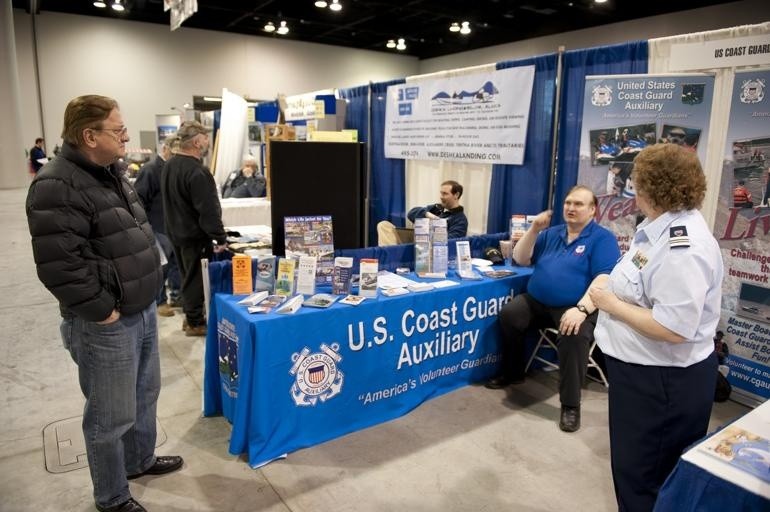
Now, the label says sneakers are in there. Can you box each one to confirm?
[158,297,208,336]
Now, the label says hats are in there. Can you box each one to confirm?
[177,120,212,141]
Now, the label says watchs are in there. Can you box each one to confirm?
[576,305,590,316]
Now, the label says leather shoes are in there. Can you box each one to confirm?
[126,455,183,480]
[96,497,146,511]
[559,405,580,432]
[485,372,525,389]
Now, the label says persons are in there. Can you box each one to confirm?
[593,131,616,155]
[222,160,266,197]
[134,135,184,317]
[618,128,630,148]
[486,184,620,432]
[634,133,655,147]
[734,181,753,208]
[160,120,227,336]
[377,181,469,246]
[589,143,724,512]
[25,95,184,511]
[667,127,687,143]
[713,331,729,365]
[606,164,623,196]
[30,138,45,172]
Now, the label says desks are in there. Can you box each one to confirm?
[653,399,770,512]
[218,197,272,226]
[224,225,272,255]
[204,261,534,470]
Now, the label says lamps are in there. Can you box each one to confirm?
[396,39,406,50]
[330,0,342,11]
[449,22,460,32]
[263,21,275,32]
[277,20,289,35]
[315,0,327,7]
[111,0,126,11]
[460,22,471,34]
[94,0,106,8]
[386,40,396,48]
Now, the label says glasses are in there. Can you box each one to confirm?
[91,126,127,135]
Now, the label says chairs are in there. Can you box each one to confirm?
[28,159,36,177]
[524,327,609,388]
